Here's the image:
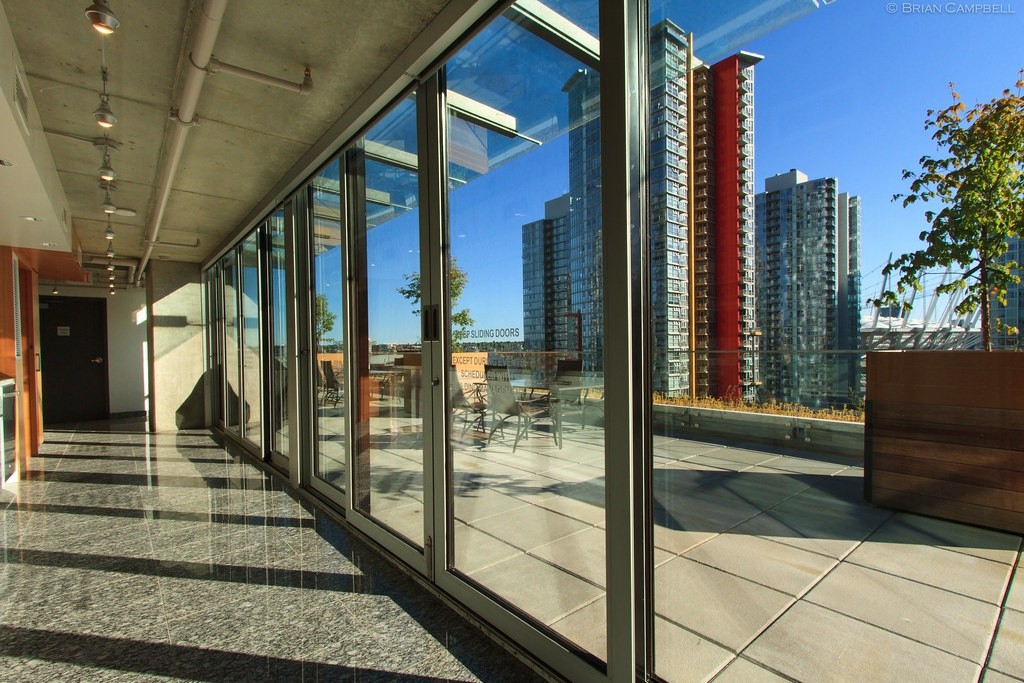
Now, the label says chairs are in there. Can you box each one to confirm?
[317,360,344,408]
[451,357,589,454]
[371,358,405,400]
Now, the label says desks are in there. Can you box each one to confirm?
[472,379,604,450]
[369,370,409,403]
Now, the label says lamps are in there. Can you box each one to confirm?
[105,258,116,295]
[51,279,59,295]
[95,135,117,181]
[100,182,117,214]
[104,239,116,258]
[102,214,116,240]
[85,0,120,35]
[92,67,118,128]
